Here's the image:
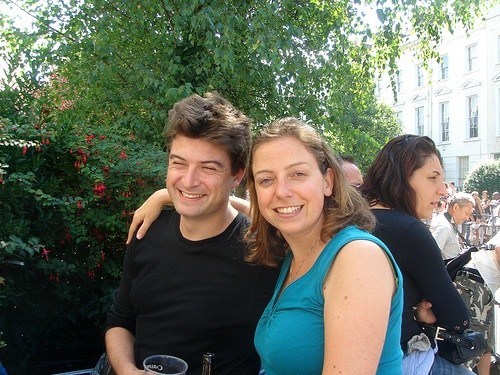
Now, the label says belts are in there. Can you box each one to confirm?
[457,271,484,284]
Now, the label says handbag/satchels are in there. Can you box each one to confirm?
[419,321,487,364]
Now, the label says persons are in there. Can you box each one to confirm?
[455,190,500,375]
[105,91,280,375]
[341,134,478,375]
[126,117,404,375]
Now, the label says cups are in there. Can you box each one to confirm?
[143,355,188,375]
